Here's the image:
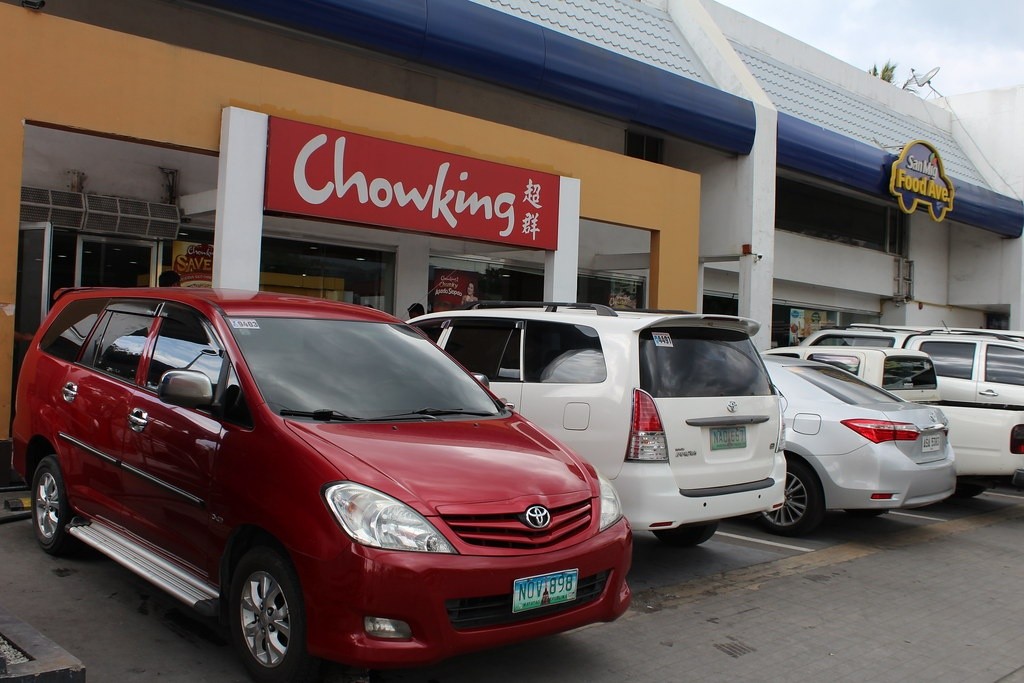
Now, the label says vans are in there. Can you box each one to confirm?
[759,346,1024,498]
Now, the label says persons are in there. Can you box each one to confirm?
[461,282,478,305]
[158,270,180,287]
[408,303,425,319]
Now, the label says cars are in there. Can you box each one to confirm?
[754,353,957,536]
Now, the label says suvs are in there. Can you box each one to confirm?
[795,324,1024,500]
[10,286,632,683]
[401,299,789,546]
